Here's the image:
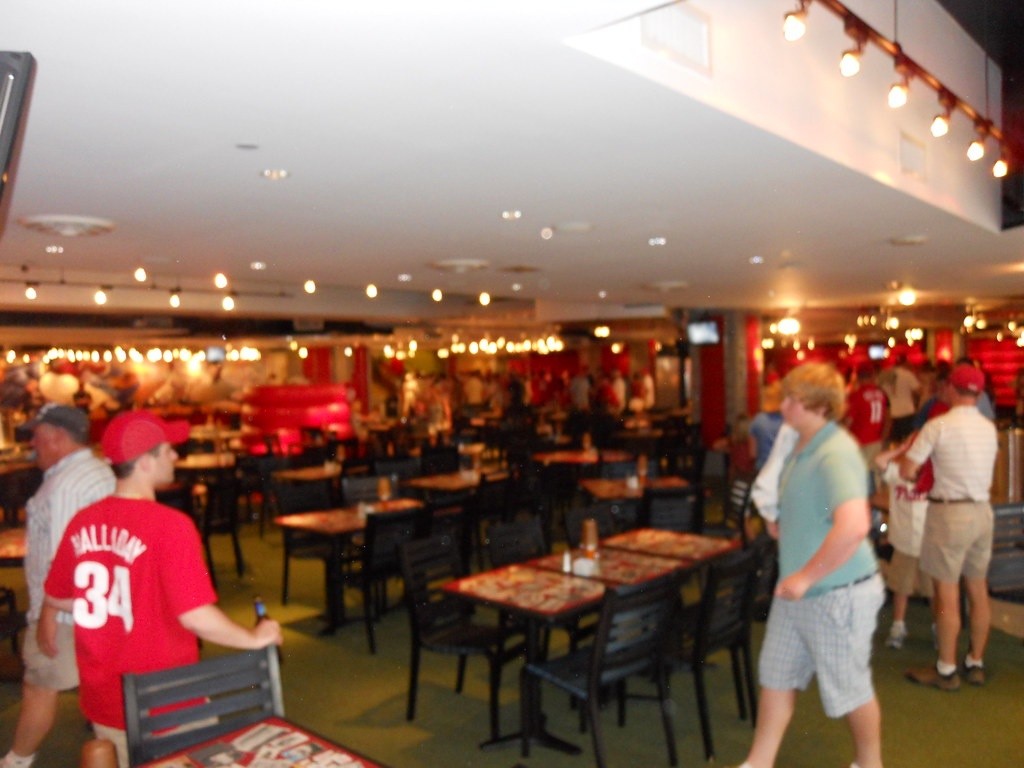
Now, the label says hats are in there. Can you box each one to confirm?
[22,401,89,439]
[856,358,874,375]
[99,407,191,465]
[950,364,985,391]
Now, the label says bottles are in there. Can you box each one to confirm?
[562,552,572,573]
[253,598,283,665]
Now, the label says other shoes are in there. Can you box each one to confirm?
[885,627,905,649]
[904,662,960,689]
[957,658,986,685]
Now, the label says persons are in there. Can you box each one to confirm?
[0,403,117,768]
[742,364,887,768]
[726,353,998,689]
[404,368,656,421]
[43,409,283,768]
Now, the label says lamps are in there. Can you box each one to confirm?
[782,0,1024,177]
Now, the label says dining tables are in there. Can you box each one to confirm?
[131,715,391,768]
[0,412,743,755]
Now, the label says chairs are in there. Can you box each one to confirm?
[120,644,284,768]
[1,412,768,768]
